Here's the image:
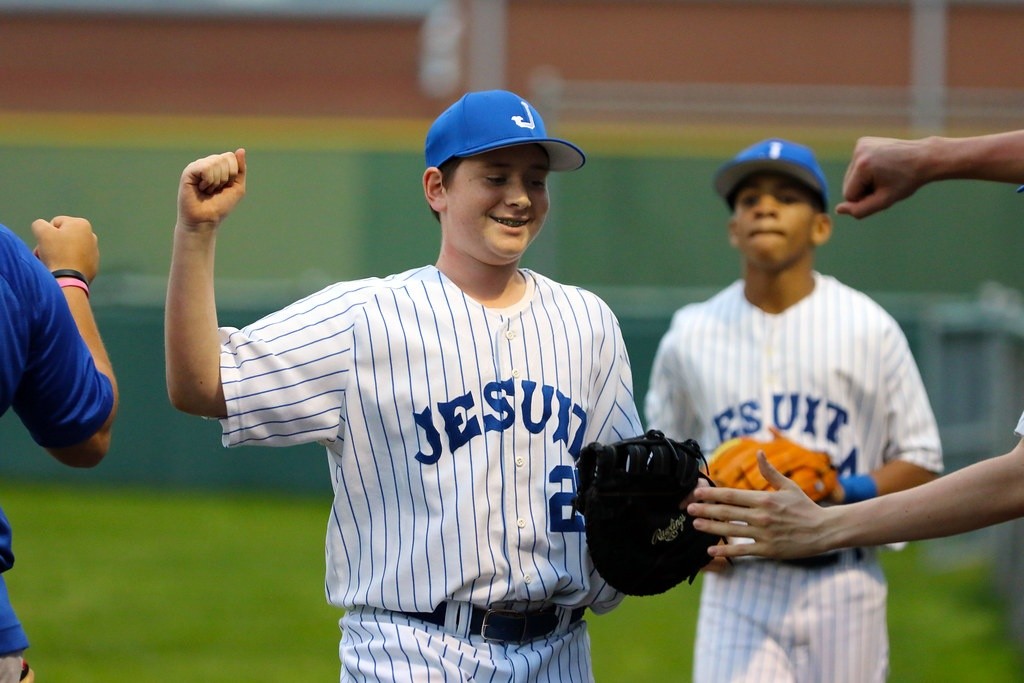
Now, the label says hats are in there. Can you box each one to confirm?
[711,140,830,200]
[425,90,585,172]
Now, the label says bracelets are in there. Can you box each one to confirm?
[840,473,878,503]
[51,268,90,301]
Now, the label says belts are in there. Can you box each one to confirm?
[395,600,587,642]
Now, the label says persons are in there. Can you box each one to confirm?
[0,213,121,683]
[837,130,1024,221]
[162,89,724,682]
[643,139,945,683]
[686,410,1023,563]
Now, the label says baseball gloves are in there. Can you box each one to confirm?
[696,425,842,505]
[574,428,727,599]
